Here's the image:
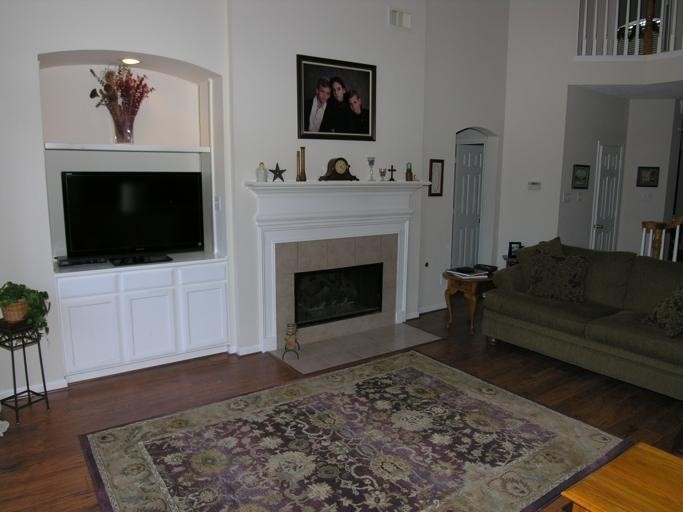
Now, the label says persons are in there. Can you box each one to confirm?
[304,76,370,133]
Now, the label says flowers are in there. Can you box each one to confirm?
[88,63,153,115]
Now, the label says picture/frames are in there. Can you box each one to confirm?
[295,53,376,141]
[571,164,590,189]
[428,159,444,196]
[636,166,660,187]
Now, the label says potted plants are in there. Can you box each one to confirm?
[0,281,50,335]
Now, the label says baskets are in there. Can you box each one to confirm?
[1,298,29,322]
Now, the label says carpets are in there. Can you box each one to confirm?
[79,349,633,512]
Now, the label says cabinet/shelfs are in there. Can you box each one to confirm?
[55,252,229,381]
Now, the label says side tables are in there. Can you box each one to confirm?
[442,268,493,337]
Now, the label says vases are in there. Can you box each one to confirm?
[108,106,138,144]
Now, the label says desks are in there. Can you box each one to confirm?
[0,318,51,424]
[560,442,683,512]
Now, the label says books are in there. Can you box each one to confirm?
[446,266,489,277]
[454,275,488,279]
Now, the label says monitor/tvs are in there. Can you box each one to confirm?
[59,170,205,265]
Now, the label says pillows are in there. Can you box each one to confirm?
[516,237,562,286]
[527,251,590,304]
[639,288,683,337]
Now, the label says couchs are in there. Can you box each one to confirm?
[480,241,683,400]
